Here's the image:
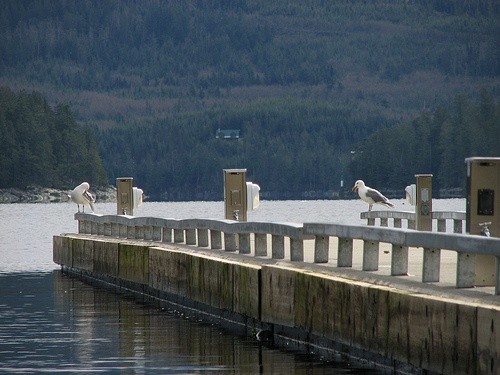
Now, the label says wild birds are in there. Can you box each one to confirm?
[351,180,395,212]
[71,182,95,213]
